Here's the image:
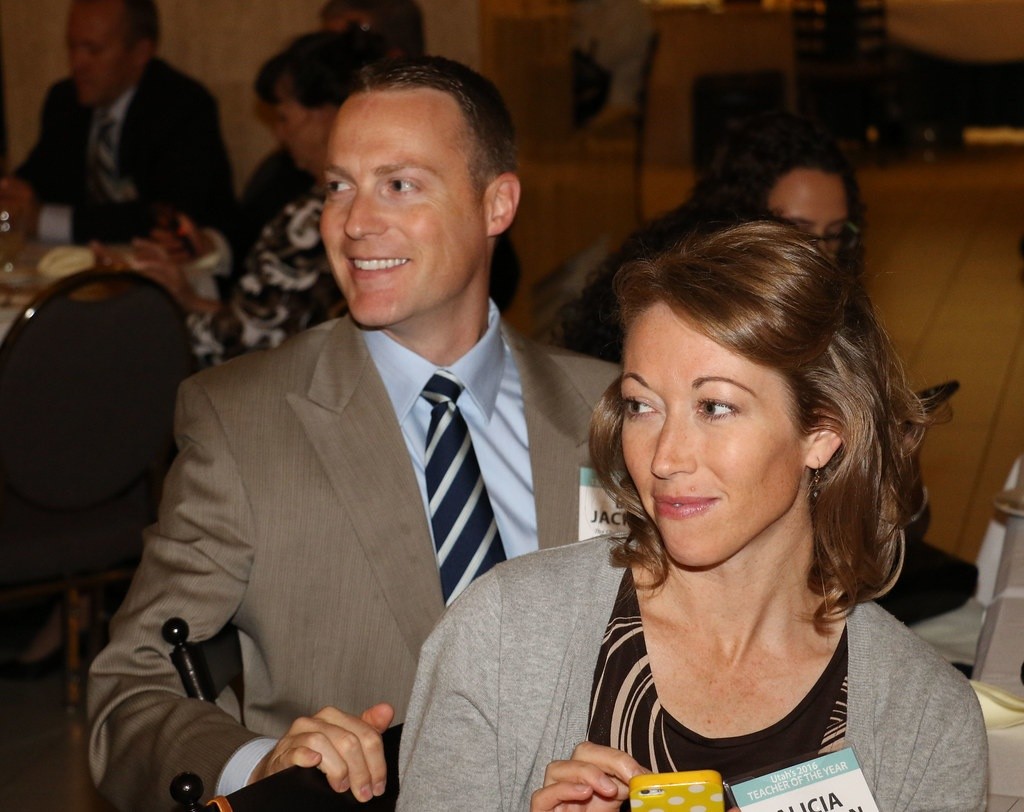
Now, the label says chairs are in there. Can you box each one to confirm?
[0,268,203,706]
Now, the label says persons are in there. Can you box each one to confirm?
[566,183,802,365]
[80,53,624,809]
[2,27,389,687]
[1,0,240,248]
[389,217,996,811]
[687,103,995,678]
[155,0,523,321]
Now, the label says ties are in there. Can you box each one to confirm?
[92,118,113,173]
[419,370,508,608]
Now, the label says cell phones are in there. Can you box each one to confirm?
[628,770,726,812]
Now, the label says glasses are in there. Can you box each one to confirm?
[785,217,859,253]
